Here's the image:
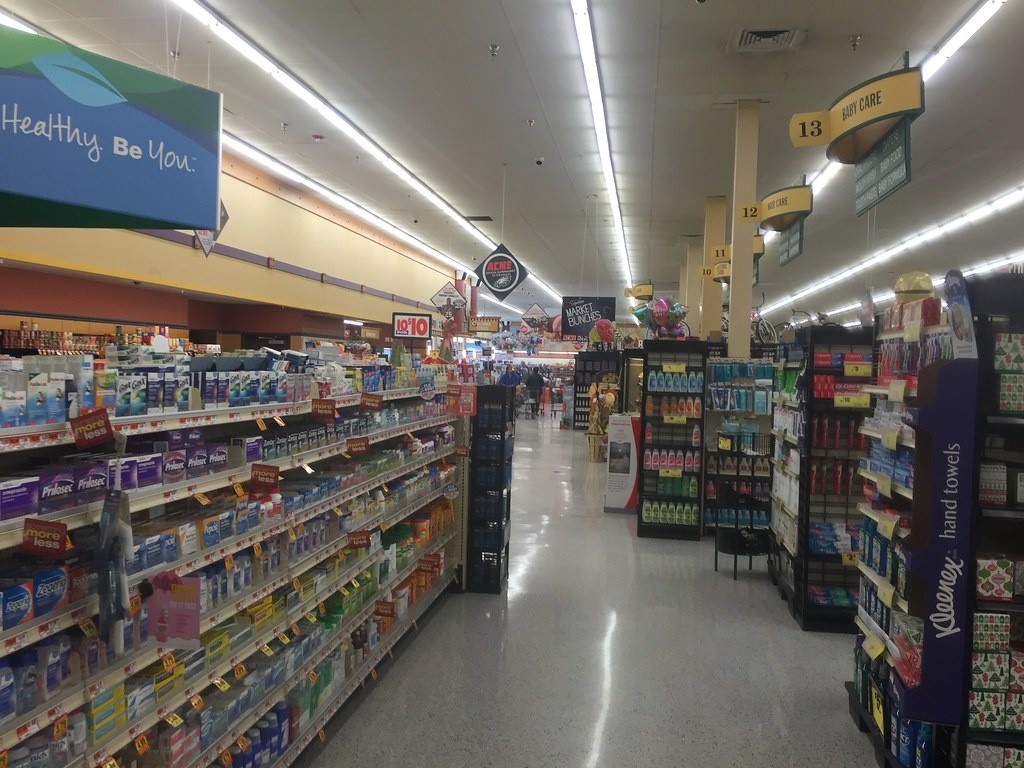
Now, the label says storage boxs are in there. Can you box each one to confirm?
[809,335,1023,768]
[1,366,410,768]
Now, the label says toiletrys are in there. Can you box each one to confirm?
[705,359,773,530]
[856,396,946,768]
[813,352,872,400]
[1,350,463,768]
[810,520,859,607]
[770,362,801,556]
[641,371,704,526]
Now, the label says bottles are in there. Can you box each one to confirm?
[707,455,771,477]
[707,481,769,502]
[705,508,768,525]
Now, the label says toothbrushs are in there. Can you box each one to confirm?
[877,335,952,374]
[808,413,869,497]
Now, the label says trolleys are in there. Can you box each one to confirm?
[526,387,546,416]
[517,391,539,419]
[550,386,562,417]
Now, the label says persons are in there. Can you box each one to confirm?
[525,367,544,419]
[521,361,529,376]
[441,298,454,314]
[499,365,520,387]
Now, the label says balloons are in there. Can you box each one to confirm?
[589,319,615,342]
[632,296,689,338]
[526,337,539,355]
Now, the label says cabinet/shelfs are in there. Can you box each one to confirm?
[623,348,645,414]
[767,324,874,631]
[636,338,731,540]
[847,272,1024,768]
[714,430,774,581]
[701,356,774,539]
[573,351,623,430]
[0,328,469,768]
[469,385,516,595]
